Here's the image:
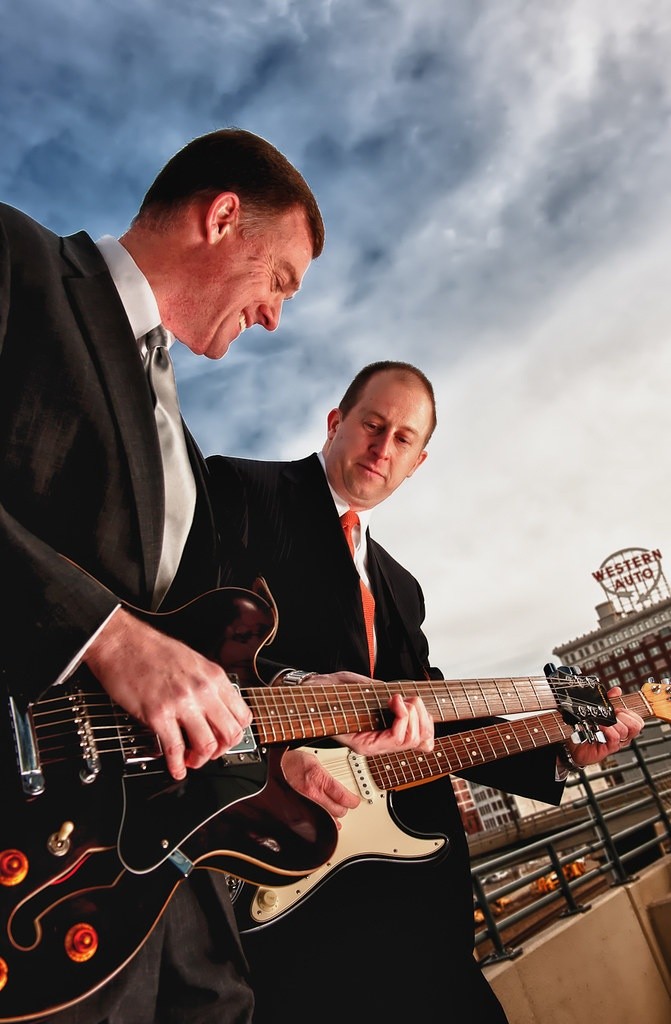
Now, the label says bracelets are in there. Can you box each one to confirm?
[281,670,319,686]
[559,743,586,773]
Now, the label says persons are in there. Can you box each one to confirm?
[208,361,645,1024]
[0,128,436,1024]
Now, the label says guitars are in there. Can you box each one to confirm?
[0,551,618,1022]
[224,676,671,937]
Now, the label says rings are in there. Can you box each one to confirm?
[620,737,628,741]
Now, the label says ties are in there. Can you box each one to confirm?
[340,510,375,678]
[143,326,198,610]
